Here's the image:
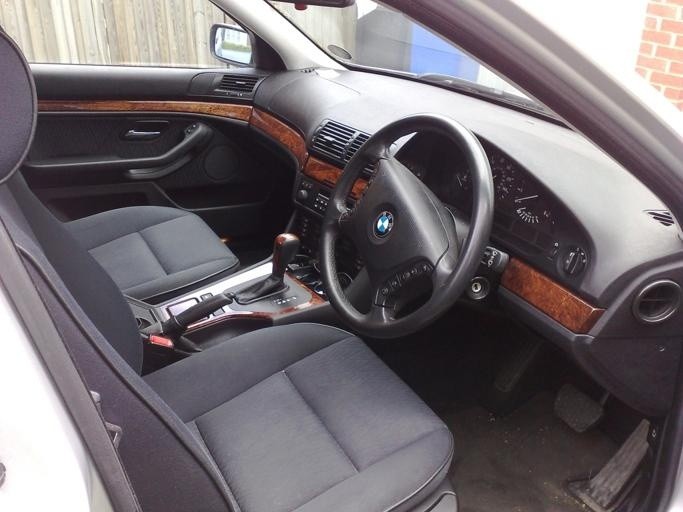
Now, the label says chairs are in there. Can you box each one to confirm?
[0,28,460,512]
[64,206,241,301]
